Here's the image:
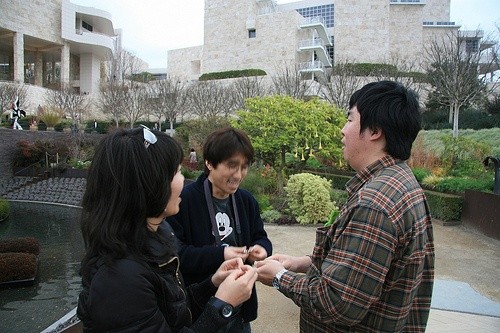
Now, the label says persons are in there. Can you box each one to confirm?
[76,80,436,333]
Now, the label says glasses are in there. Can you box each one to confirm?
[138,124,158,149]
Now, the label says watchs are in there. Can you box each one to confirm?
[208,296,234,319]
[272,269,288,291]
